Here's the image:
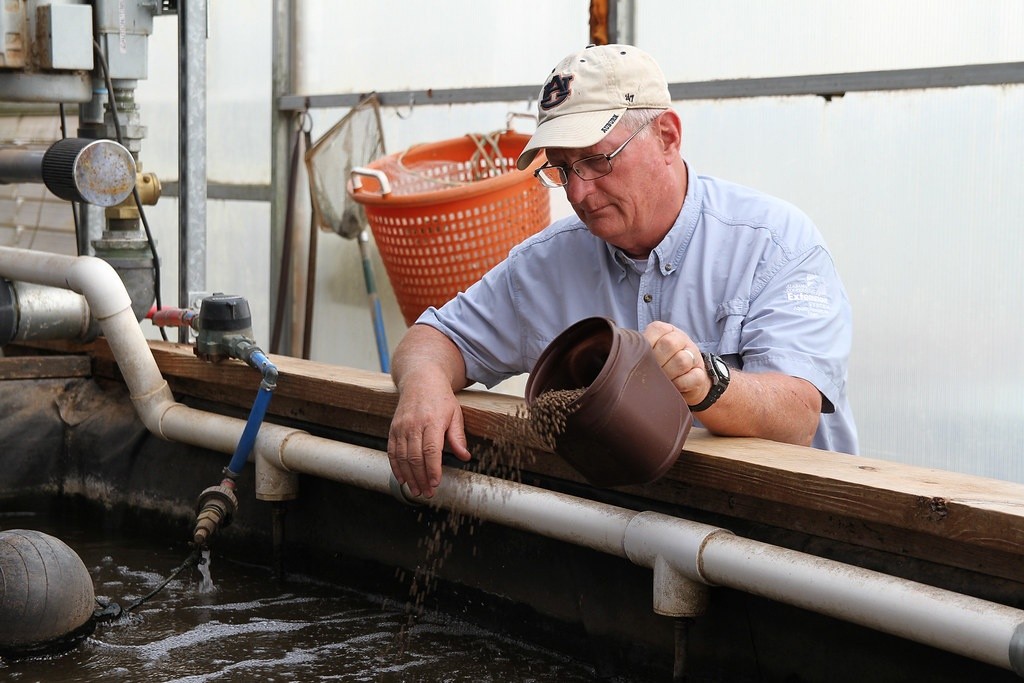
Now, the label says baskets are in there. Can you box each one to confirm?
[348,112,551,329]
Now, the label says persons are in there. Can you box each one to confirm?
[387,44,858,498]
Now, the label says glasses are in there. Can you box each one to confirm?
[533,118,655,189]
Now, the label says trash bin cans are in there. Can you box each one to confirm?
[346,131,551,329]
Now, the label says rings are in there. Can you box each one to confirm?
[685,350,694,366]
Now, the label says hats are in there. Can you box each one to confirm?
[517,43,670,171]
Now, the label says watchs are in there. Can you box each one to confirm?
[688,352,731,412]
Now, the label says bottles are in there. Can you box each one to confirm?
[523,316,694,492]
